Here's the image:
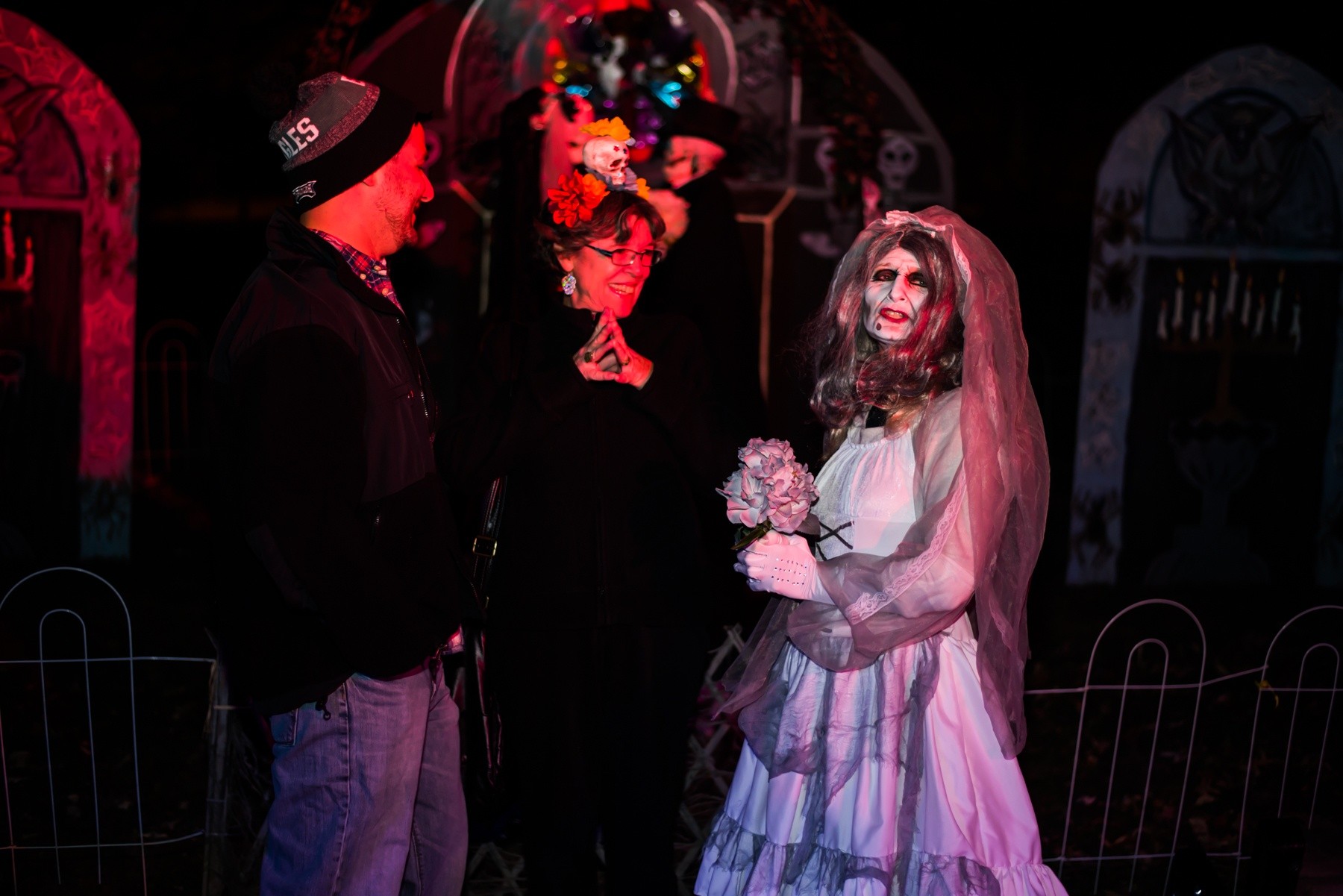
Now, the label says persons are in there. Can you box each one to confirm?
[492,170,732,889]
[688,208,1062,896]
[232,77,476,896]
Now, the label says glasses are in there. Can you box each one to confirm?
[582,242,662,267]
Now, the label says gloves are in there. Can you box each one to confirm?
[734,529,845,605]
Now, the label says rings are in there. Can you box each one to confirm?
[582,351,595,363]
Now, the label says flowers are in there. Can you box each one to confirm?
[717,434,826,552]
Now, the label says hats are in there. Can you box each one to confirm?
[268,72,416,222]
